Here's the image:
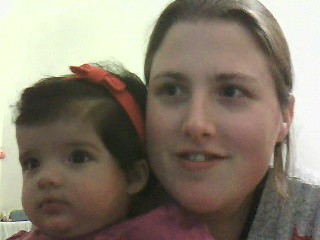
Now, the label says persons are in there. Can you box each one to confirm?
[143,0,320,240]
[4,63,213,240]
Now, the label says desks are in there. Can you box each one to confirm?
[0,221,31,240]
